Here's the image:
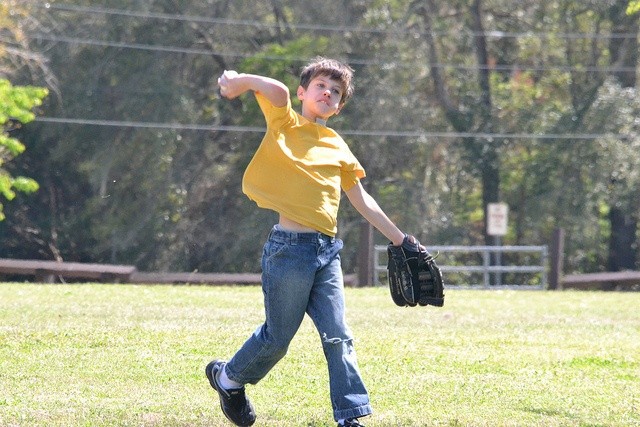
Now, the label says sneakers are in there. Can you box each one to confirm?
[206,360,256,427]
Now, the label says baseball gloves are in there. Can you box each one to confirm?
[387,233,445,307]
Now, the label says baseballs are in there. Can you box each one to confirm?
[227,70,238,77]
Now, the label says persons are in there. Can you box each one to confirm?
[204,56,446,427]
